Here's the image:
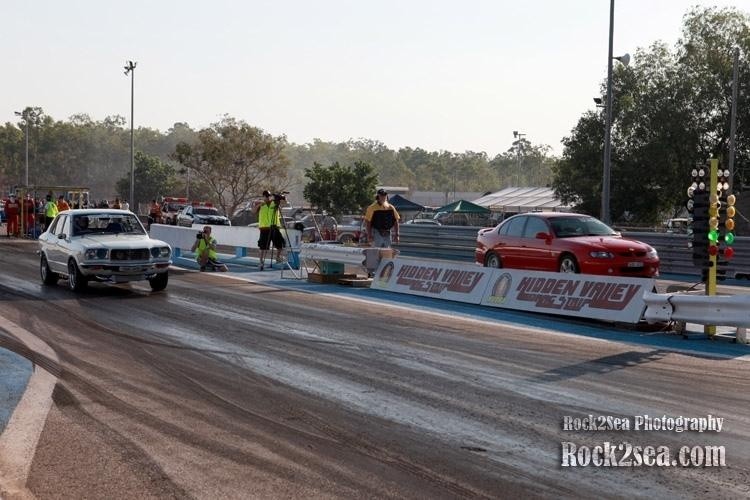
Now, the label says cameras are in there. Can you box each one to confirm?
[196,230,206,239]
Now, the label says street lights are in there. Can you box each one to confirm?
[512,130,526,188]
[592,97,614,226]
[124,60,136,213]
[15,110,44,193]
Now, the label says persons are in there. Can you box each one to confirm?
[251,190,285,271]
[4,190,130,237]
[149,199,159,223]
[365,189,400,278]
[192,226,228,272]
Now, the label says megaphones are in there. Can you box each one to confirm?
[612,53,630,66]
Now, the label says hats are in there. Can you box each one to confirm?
[263,189,272,197]
[377,188,387,196]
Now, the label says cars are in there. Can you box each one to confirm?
[662,218,693,235]
[0,197,9,226]
[36,208,173,294]
[474,211,660,280]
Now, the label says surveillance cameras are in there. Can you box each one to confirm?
[593,96,603,107]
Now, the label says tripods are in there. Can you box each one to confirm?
[260,209,297,268]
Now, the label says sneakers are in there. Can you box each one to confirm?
[368,271,377,278]
